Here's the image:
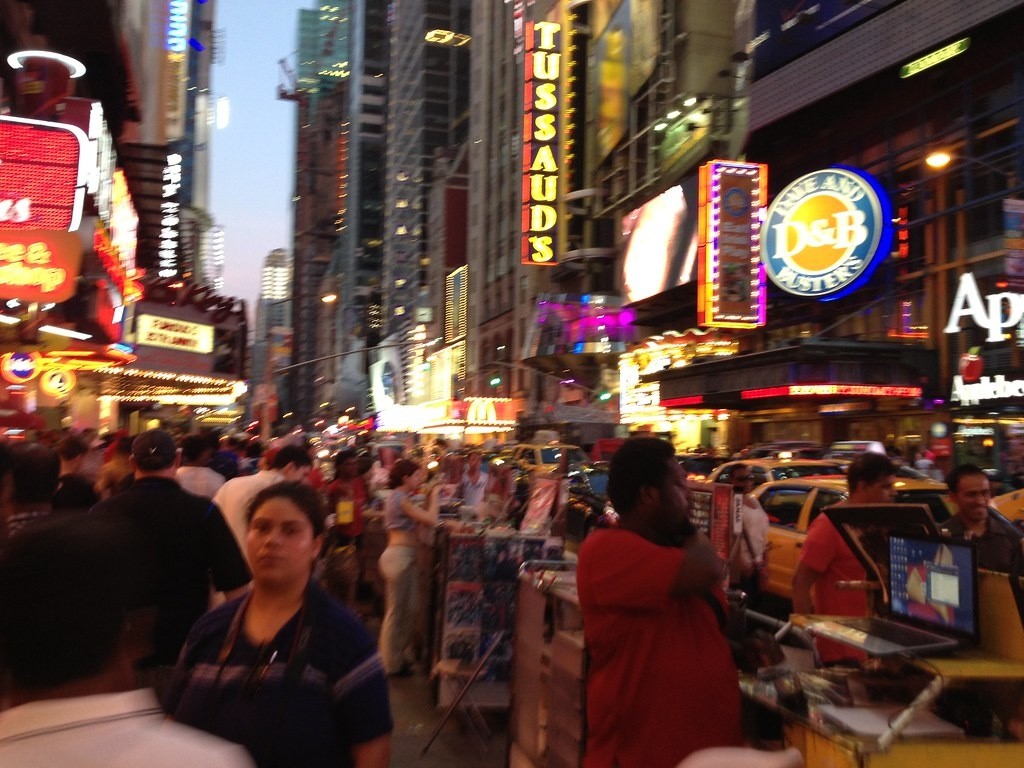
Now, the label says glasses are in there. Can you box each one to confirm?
[734,474,755,482]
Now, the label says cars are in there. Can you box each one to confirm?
[368,441,1024,648]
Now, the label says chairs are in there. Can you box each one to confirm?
[422,630,504,753]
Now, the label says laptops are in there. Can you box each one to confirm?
[807,530,979,656]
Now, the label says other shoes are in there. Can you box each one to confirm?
[389,666,415,678]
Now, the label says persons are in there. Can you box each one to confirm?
[0,427,1023,768]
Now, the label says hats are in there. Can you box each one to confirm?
[132,429,176,465]
